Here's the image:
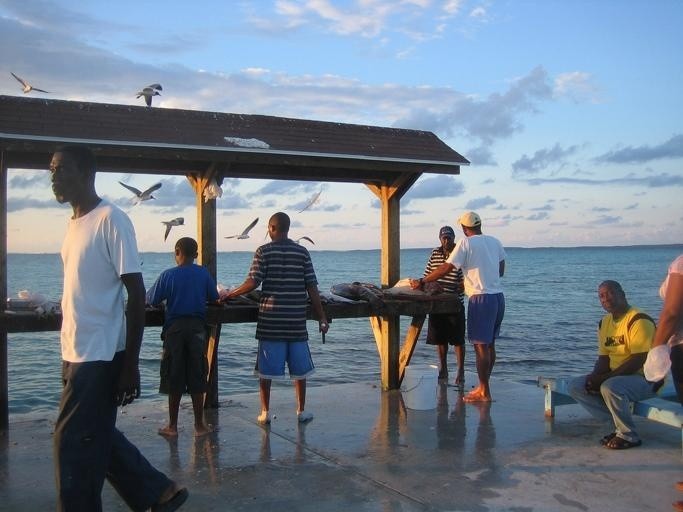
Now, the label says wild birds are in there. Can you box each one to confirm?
[10,71,50,94]
[118,181,162,205]
[224,218,259,239]
[137,84,162,107]
[160,218,184,241]
[300,188,323,212]
[293,236,314,245]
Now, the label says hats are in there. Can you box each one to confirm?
[457,212,483,228]
[439,228,455,239]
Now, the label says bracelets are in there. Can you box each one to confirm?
[420,278,426,287]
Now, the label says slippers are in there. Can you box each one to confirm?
[600,433,641,448]
[151,488,189,512]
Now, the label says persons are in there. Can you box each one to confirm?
[568,279,666,450]
[146,237,222,438]
[214,211,330,425]
[420,225,467,384]
[44,141,194,512]
[647,252,683,512]
[409,209,508,404]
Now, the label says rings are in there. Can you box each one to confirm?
[130,393,135,397]
[124,395,130,400]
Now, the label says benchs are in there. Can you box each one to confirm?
[537,374,683,429]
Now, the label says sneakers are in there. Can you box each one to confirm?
[258,413,271,424]
[297,411,315,421]
